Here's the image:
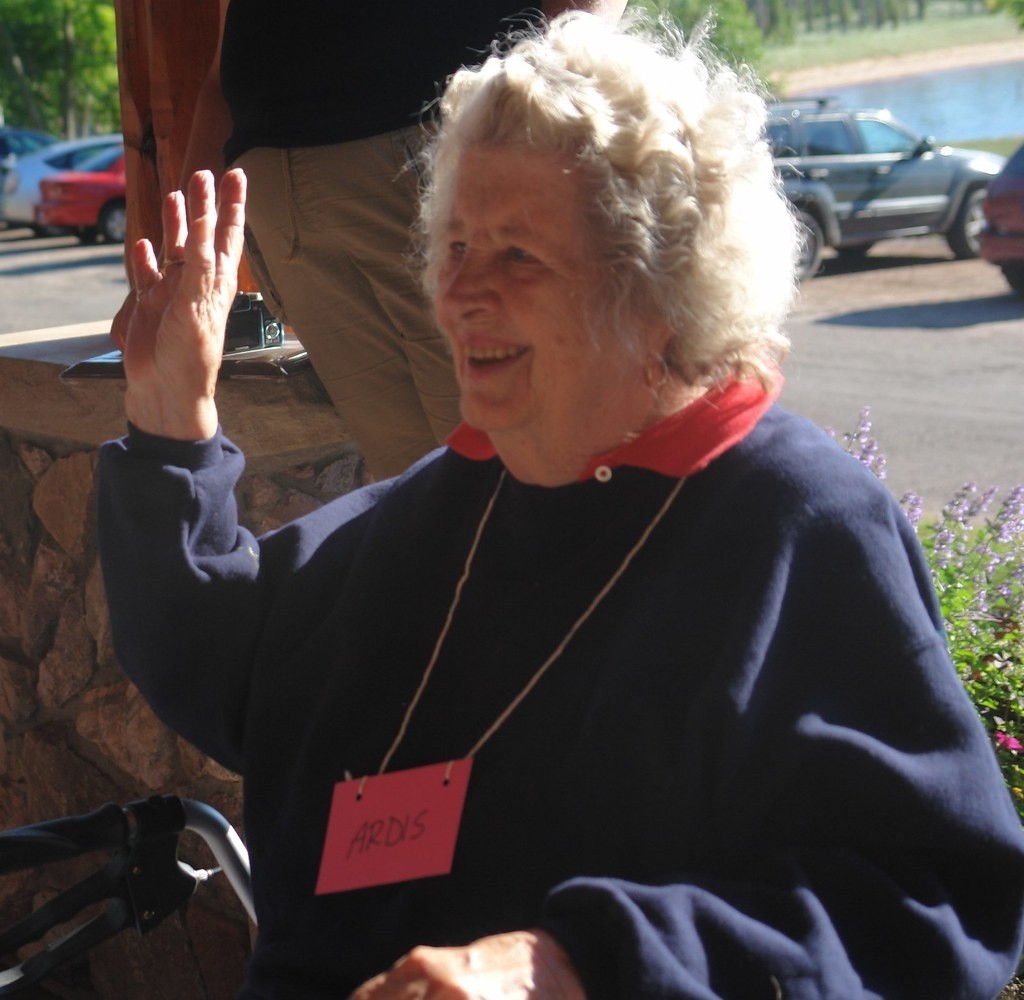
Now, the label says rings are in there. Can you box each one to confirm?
[163,257,186,267]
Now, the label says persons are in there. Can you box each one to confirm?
[98,9,1024,1000]
[181,0,628,485]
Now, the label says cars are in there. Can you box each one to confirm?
[0,134,124,239]
[975,142,1024,298]
[33,143,128,244]
[0,126,66,179]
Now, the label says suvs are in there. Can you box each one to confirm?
[761,92,1011,283]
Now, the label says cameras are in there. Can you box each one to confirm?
[222,291,284,355]
